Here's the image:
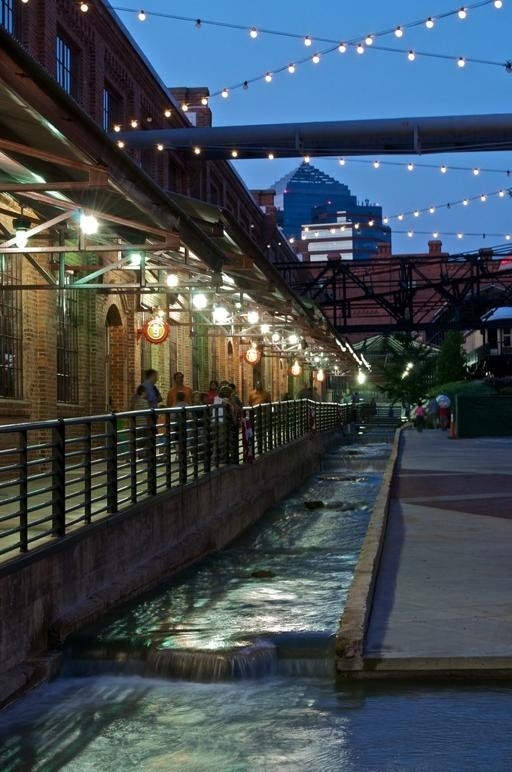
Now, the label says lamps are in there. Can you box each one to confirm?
[9,195,35,254]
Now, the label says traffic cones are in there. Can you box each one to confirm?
[447,412,456,439]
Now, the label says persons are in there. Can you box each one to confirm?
[284,387,377,418]
[415,394,452,433]
[249,380,271,406]
[131,369,243,466]
